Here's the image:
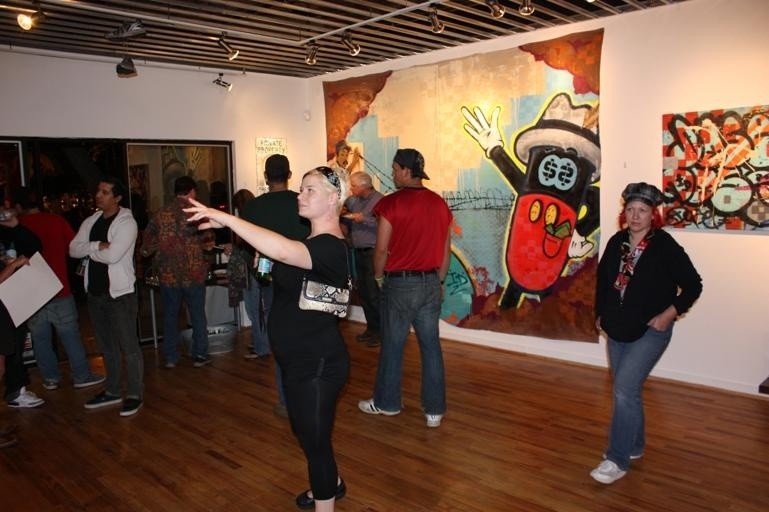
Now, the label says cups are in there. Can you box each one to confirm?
[6,249,17,260]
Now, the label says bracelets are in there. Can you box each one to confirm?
[374,273,385,283]
[440,281,444,285]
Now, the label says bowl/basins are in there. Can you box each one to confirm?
[181,325,236,356]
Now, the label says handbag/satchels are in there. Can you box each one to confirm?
[298,237,354,319]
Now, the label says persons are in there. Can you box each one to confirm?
[590,182,703,486]
[241,155,311,418]
[329,139,362,205]
[0,176,145,416]
[224,189,272,359]
[358,148,453,426]
[182,167,350,512]
[341,171,386,346]
[140,176,215,369]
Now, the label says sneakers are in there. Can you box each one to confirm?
[243,351,262,361]
[7,386,46,410]
[118,398,144,417]
[41,377,60,390]
[366,337,383,348]
[423,412,445,428]
[589,458,628,485]
[357,395,402,417]
[355,332,378,343]
[191,354,214,369]
[162,357,177,369]
[295,477,347,509]
[71,371,108,389]
[83,391,124,411]
[603,449,645,461]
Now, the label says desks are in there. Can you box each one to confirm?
[143,266,250,353]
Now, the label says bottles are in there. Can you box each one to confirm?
[256,253,271,281]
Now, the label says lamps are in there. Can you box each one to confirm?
[295,0,541,67]
[14,2,240,92]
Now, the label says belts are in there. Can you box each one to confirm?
[382,268,439,277]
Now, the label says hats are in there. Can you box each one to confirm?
[618,181,667,209]
[392,147,430,181]
